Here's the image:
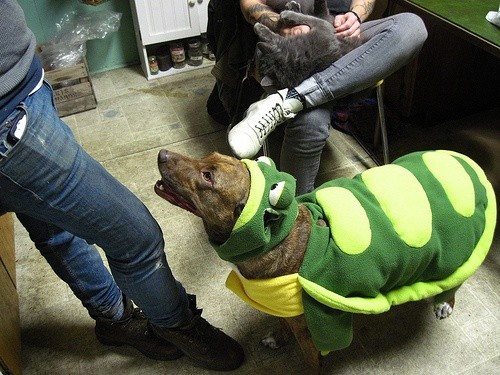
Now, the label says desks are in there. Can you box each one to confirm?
[392,0,500,130]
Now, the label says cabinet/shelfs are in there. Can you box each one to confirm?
[130,0,212,82]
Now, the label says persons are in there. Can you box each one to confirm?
[228,0,427,194]
[0,0,245,371]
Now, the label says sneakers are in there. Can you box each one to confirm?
[148,293,243,369]
[90,291,182,359]
[227,93,303,161]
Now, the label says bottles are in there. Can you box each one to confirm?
[148,56,159,75]
[158,52,172,71]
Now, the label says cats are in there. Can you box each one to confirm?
[253,0,374,95]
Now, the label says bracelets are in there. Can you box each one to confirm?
[348,10,361,24]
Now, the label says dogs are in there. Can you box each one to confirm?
[153,147,497,375]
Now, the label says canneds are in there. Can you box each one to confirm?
[148,38,203,75]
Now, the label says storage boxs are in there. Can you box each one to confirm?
[34,40,97,119]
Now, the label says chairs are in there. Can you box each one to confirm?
[206,0,390,167]
